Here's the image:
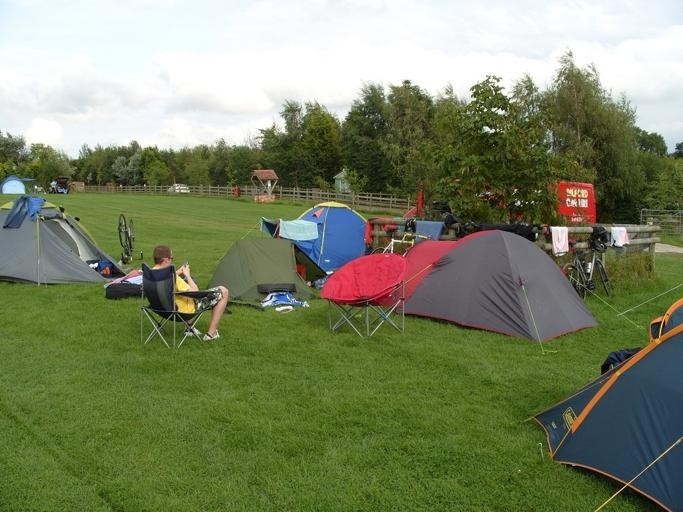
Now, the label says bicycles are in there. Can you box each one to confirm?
[560,231,612,300]
[366,217,431,257]
[114,213,144,264]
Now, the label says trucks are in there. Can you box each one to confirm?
[402,176,596,225]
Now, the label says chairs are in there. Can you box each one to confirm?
[317,251,406,337]
[135,260,221,348]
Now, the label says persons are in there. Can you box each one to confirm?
[33,179,58,194]
[151,245,229,342]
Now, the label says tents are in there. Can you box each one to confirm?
[262,202,374,274]
[207,239,329,311]
[0,194,128,287]
[523,297,682,512]
[0,175,25,195]
[402,231,595,342]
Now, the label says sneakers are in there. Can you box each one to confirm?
[202,330,219,341]
[184,327,202,337]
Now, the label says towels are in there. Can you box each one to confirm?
[549,224,570,257]
[610,226,629,247]
[259,215,319,242]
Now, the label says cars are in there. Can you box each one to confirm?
[167,183,190,195]
[47,176,69,194]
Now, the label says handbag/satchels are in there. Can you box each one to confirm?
[105,280,144,299]
